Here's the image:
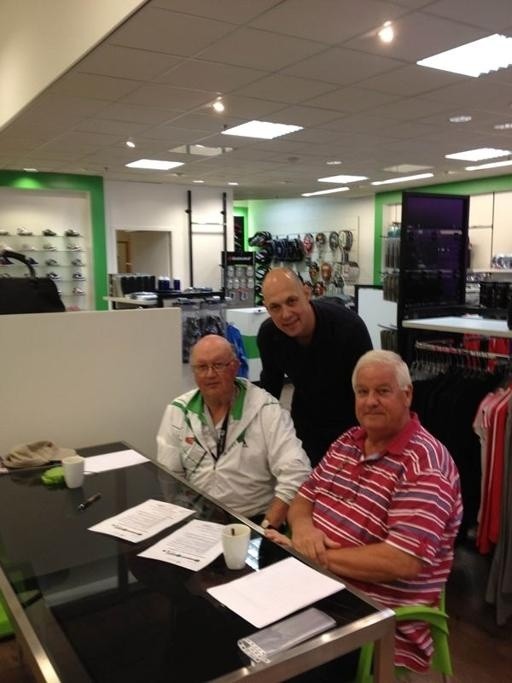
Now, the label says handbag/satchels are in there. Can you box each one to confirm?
[1,250,65,313]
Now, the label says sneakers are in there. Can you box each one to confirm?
[0,229,86,294]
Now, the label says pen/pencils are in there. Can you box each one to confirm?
[78,492,102,510]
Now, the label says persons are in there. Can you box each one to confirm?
[247,265,378,469]
[153,334,315,533]
[264,348,468,673]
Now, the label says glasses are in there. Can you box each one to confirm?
[190,358,234,370]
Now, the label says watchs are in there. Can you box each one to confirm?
[260,516,278,530]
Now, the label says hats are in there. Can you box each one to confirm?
[2,440,76,469]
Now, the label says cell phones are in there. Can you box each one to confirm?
[237,607,336,663]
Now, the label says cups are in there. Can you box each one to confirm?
[158,279,180,291]
[62,455,85,488]
[221,523,250,569]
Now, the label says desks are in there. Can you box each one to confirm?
[0,442,401,683]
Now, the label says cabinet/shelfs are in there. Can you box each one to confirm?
[0,229,87,300]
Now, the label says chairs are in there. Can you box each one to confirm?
[355,573,456,683]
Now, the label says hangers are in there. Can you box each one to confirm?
[409,335,512,381]
